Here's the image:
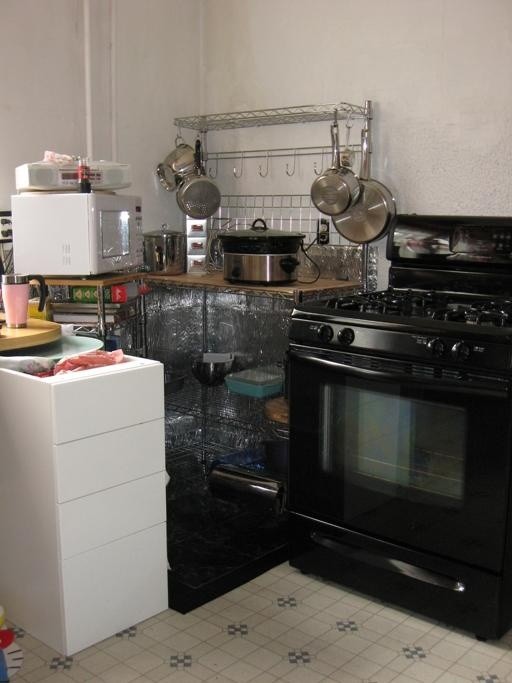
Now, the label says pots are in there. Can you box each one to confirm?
[155,135,221,220]
[217,218,307,286]
[142,222,188,277]
[310,121,397,244]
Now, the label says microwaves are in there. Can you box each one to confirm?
[11,192,143,281]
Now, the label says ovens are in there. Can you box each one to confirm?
[283,318,512,573]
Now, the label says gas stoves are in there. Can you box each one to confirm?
[298,287,511,335]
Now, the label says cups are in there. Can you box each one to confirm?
[1,273,46,329]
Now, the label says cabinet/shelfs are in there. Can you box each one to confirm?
[135,289,364,496]
[29,272,146,360]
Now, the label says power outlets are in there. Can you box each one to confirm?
[318,217,329,244]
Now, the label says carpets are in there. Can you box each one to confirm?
[161,470,288,618]
